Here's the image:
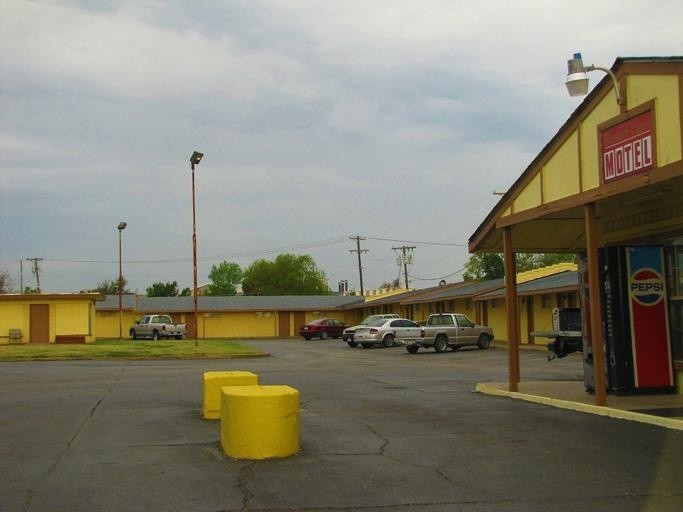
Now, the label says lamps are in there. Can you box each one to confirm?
[565,50,625,110]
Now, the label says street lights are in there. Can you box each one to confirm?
[117,219,128,338]
[188,149,205,349]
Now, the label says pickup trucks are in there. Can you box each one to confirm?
[128,313,188,341]
[394,314,497,355]
[342,312,428,349]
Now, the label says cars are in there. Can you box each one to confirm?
[298,318,350,341]
[353,317,426,348]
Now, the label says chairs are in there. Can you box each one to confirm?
[8,328,23,345]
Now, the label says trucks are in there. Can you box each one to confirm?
[529,303,585,359]
